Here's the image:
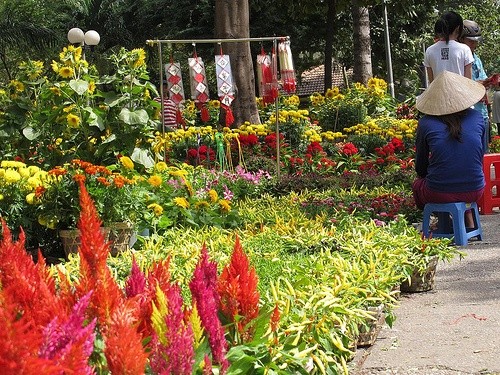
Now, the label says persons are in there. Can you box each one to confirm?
[492,82,500,136]
[152,80,178,129]
[411,68,489,241]
[422,10,476,85]
[457,18,500,141]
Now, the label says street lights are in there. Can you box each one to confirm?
[68,28,100,107]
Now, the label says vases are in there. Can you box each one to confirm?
[60,222,133,261]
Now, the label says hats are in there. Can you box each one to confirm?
[416,71,486,116]
[461,20,482,41]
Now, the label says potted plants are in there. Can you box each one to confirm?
[395,227,466,293]
[324,255,403,347]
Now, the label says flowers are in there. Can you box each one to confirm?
[35,159,140,229]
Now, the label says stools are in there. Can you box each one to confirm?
[423,202,483,246]
[478,153,500,215]
